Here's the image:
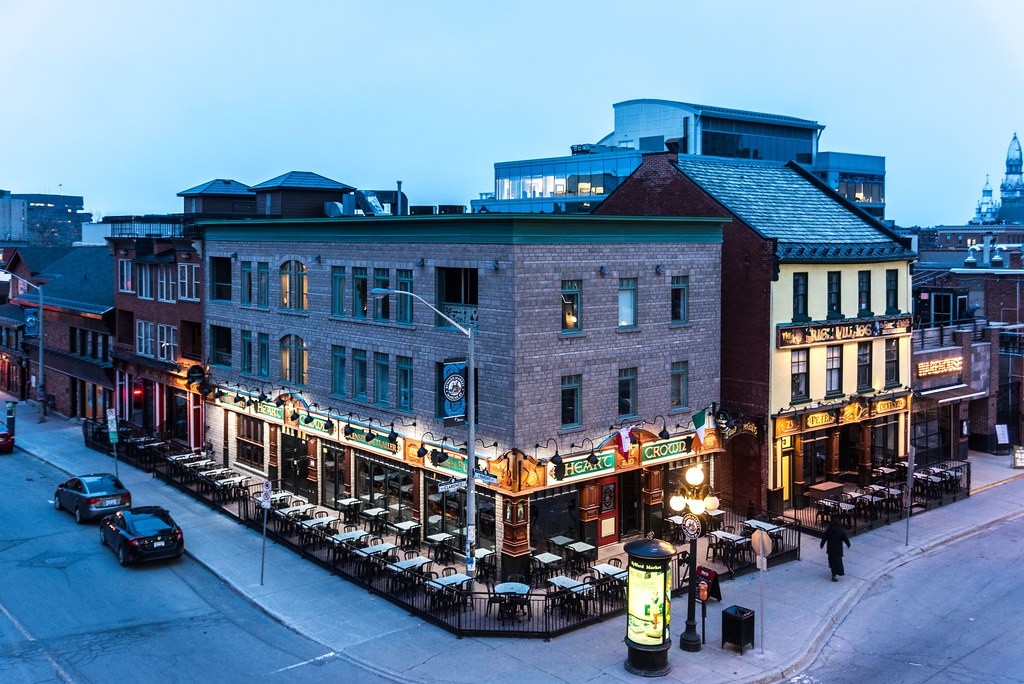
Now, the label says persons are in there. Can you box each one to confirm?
[820,517,849,581]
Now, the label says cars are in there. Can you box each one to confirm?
[99,504,185,567]
[54,473,132,524]
[0,420,15,454]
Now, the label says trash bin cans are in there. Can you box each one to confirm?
[722,605,755,655]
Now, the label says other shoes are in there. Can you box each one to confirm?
[832,577,838,582]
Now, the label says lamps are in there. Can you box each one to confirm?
[610,418,640,445]
[310,254,323,263]
[599,264,609,276]
[228,248,238,264]
[203,377,498,464]
[795,314,899,337]
[653,263,666,276]
[640,413,671,439]
[707,407,741,429]
[569,435,604,463]
[534,437,565,467]
[778,381,925,418]
[488,258,500,271]
[414,255,423,270]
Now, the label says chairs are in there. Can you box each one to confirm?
[813,462,963,528]
[139,440,785,626]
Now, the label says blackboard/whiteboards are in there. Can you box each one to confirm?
[994,424,1010,444]
[696,566,722,601]
[1010,445,1024,467]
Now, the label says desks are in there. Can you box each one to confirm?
[815,461,964,522]
[130,439,795,614]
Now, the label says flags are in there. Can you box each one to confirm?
[691,407,709,453]
[614,424,642,460]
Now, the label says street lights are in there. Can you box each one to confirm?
[370,288,477,579]
[665,464,720,653]
[0,269,48,423]
